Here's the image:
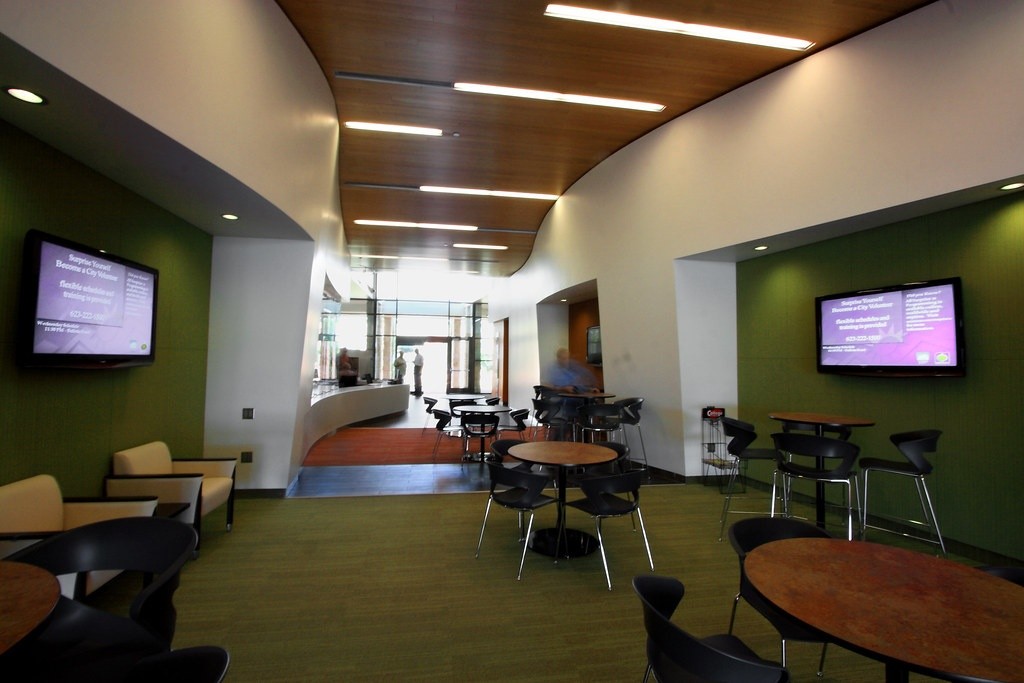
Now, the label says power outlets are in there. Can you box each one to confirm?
[243,408,253,419]
[241,452,252,463]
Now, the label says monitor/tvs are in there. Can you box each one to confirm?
[16,228,159,370]
[816,276,970,378]
[586,325,603,365]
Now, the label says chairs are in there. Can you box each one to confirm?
[423,386,1024,683]
[0,515,198,648]
[123,645,228,683]
[106,440,237,558]
[0,475,159,600]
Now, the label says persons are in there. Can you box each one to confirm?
[337,354,358,388]
[410,348,424,396]
[545,346,600,418]
[394,351,407,380]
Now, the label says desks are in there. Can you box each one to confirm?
[507,441,620,559]
[451,405,511,462]
[557,392,616,443]
[0,561,62,657]
[439,394,485,433]
[765,410,878,523]
[744,537,1024,683]
